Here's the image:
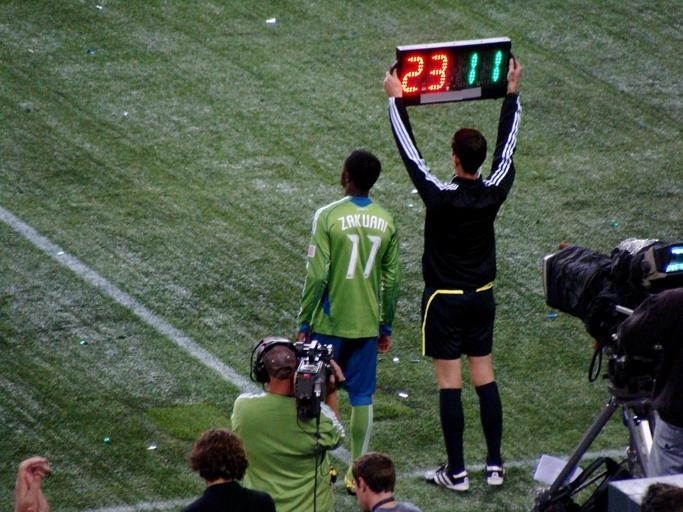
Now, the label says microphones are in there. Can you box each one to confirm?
[249,339,263,382]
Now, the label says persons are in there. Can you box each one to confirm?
[351,453,422,512]
[616,288,683,478]
[15,456,49,512]
[383,58,523,491]
[180,427,276,512]
[229,337,347,512]
[295,150,400,495]
[641,481,683,512]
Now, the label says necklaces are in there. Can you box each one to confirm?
[370,497,394,508]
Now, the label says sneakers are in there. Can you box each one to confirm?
[425,464,470,491]
[343,475,355,496]
[485,465,506,487]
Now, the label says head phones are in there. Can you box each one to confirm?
[254,338,301,383]
